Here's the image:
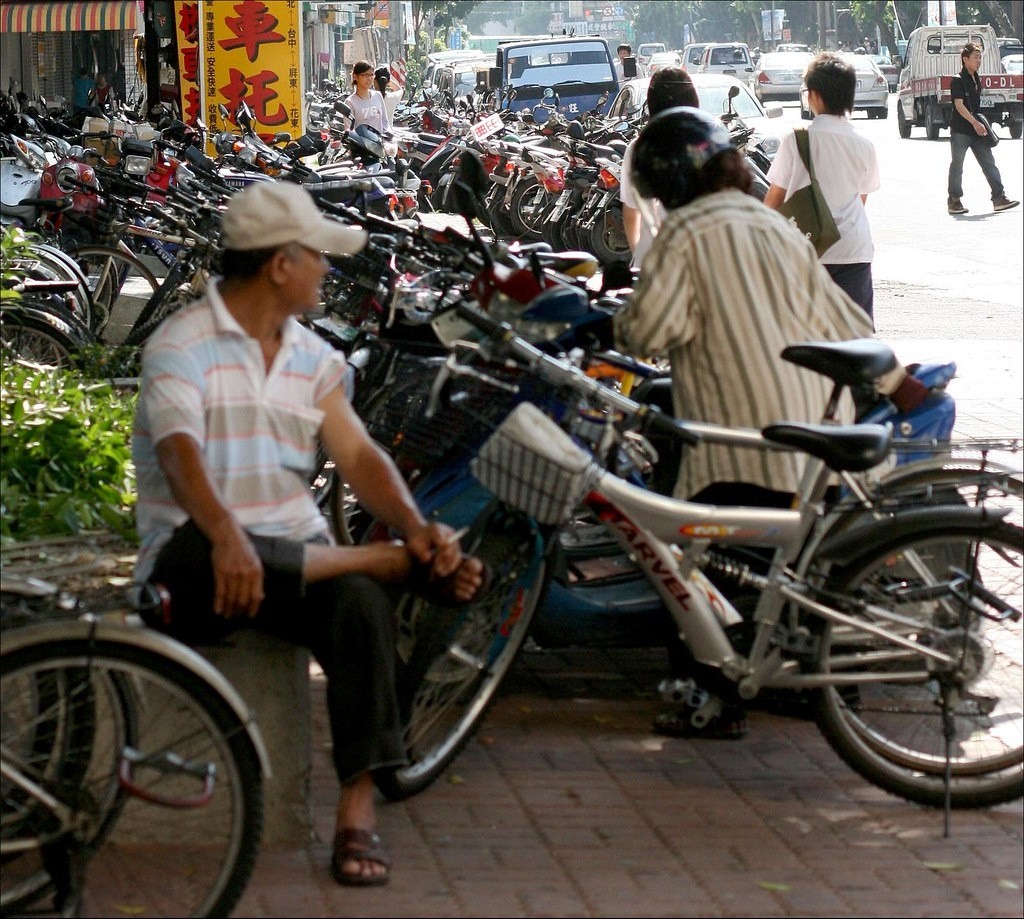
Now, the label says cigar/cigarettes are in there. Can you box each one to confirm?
[432,526,469,554]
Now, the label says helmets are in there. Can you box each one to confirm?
[374,67,390,81]
[631,111,736,199]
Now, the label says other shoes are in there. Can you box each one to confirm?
[780,692,864,719]
[649,708,747,740]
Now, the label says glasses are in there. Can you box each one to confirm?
[359,73,373,76]
[802,88,813,97]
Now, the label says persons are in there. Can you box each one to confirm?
[612,106,928,739]
[946,43,1021,213]
[614,44,645,91]
[619,67,698,269]
[132,182,492,888]
[70,68,115,116]
[343,61,392,133]
[373,66,404,130]
[761,52,881,320]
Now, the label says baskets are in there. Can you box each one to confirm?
[461,398,607,526]
[368,381,473,465]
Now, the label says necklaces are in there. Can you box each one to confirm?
[356,92,371,99]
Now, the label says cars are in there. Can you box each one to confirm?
[426,50,497,104]
[1001,53,1023,76]
[609,73,784,160]
[867,54,898,93]
[644,53,682,75]
[744,51,817,105]
[798,51,890,119]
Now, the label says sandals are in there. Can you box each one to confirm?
[331,828,392,887]
[397,552,494,610]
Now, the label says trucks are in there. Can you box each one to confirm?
[891,24,1024,140]
[996,37,1023,58]
[489,33,637,118]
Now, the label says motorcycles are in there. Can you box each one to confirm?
[0,79,961,688]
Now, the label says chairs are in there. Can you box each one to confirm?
[711,54,720,65]
[730,52,745,63]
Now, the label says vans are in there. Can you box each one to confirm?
[682,43,713,73]
[637,43,665,75]
[693,43,755,83]
[777,44,809,53]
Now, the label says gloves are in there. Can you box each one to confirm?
[890,371,929,413]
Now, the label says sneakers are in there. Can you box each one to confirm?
[948,197,970,213]
[994,197,1020,211]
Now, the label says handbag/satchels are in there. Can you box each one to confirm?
[970,112,999,147]
[775,128,841,259]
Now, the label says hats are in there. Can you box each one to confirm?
[221,178,369,259]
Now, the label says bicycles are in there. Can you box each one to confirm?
[0,158,1024,919]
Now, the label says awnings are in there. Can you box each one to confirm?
[0,1,137,33]
